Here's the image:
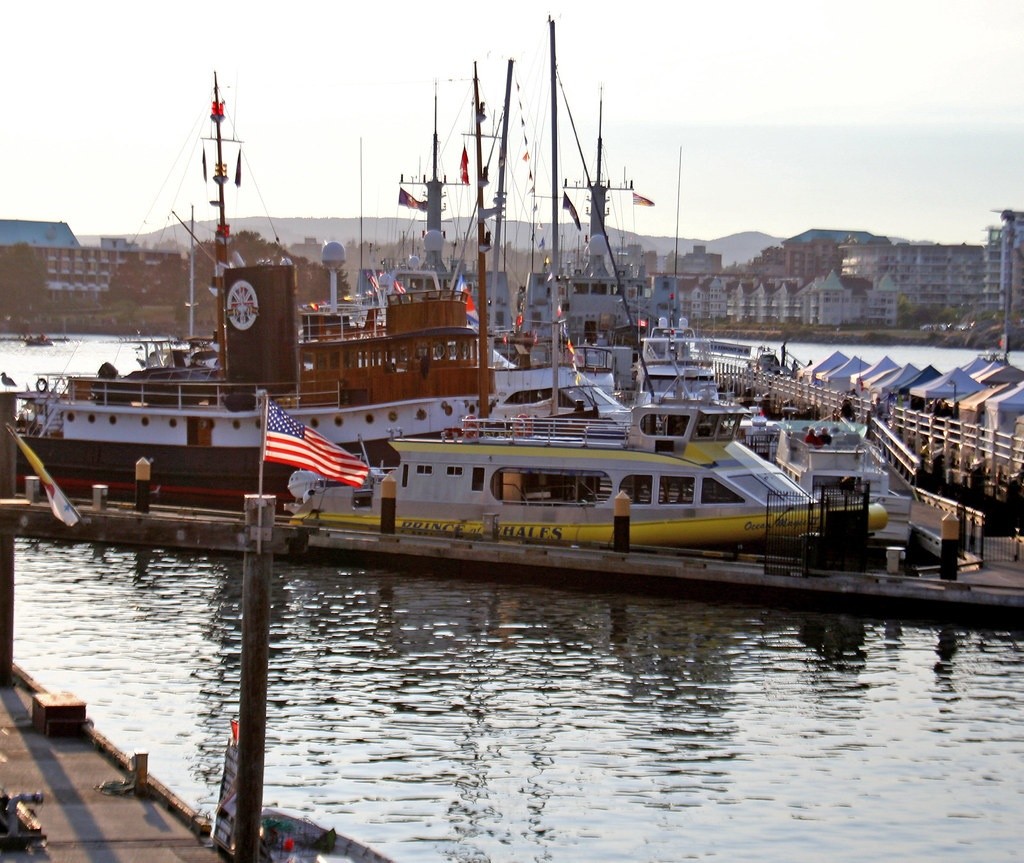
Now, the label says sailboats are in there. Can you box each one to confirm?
[1,13,910,558]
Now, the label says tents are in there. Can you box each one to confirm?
[802,350,1024,464]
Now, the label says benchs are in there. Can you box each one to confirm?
[31,691,87,737]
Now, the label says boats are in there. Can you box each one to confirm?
[258,809,396,863]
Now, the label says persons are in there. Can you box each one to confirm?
[869,397,882,419]
[839,399,856,422]
[941,403,953,418]
[804,428,823,449]
[818,427,832,445]
[758,393,771,419]
[781,342,787,367]
[851,389,857,395]
[791,360,797,378]
[926,404,933,413]
[932,400,943,417]
[783,399,794,421]
[742,389,752,407]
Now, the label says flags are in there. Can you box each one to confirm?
[460,146,470,186]
[562,191,581,231]
[633,193,655,206]
[398,187,428,213]
[453,272,480,324]
[263,397,370,489]
[393,279,406,295]
[362,269,378,292]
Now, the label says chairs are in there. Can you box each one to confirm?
[316,308,379,342]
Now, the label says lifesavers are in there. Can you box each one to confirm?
[463,415,479,438]
[35,377,48,393]
[513,414,531,437]
